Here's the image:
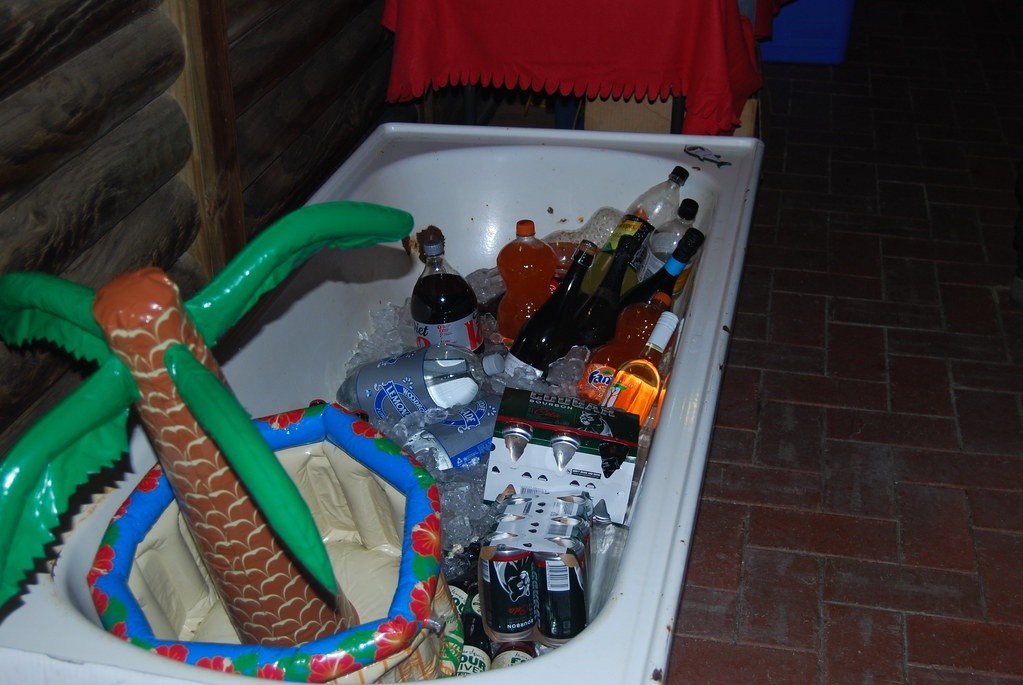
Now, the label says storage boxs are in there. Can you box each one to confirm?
[737,0,854,65]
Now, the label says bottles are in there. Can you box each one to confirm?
[335,165,708,677]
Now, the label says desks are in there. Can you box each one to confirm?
[380,0,793,138]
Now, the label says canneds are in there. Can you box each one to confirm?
[479,496,593,648]
